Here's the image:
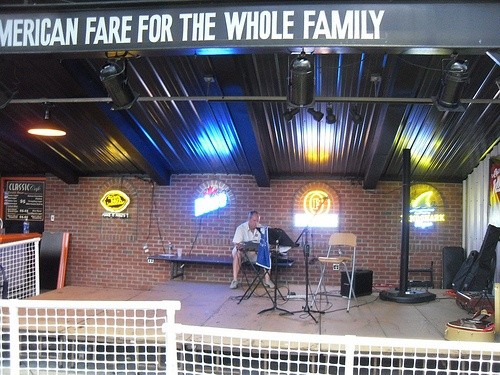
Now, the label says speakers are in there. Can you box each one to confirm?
[442,246,464,289]
[340,269,372,296]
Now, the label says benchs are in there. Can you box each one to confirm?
[148,253,296,288]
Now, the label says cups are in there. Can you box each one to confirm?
[177,248,183,257]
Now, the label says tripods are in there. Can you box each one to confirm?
[279,201,326,323]
[255,227,297,316]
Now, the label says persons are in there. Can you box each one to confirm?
[229,211,276,289]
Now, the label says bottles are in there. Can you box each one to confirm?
[23,218,30,234]
[168,242,173,255]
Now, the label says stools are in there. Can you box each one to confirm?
[312,232,357,313]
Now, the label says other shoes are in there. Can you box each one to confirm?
[263,278,275,289]
[230,280,240,289]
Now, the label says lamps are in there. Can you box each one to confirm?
[348,104,363,123]
[282,106,300,121]
[431,49,472,113]
[324,103,336,124]
[283,47,315,109]
[26,102,66,138]
[100,51,141,113]
[307,107,324,123]
